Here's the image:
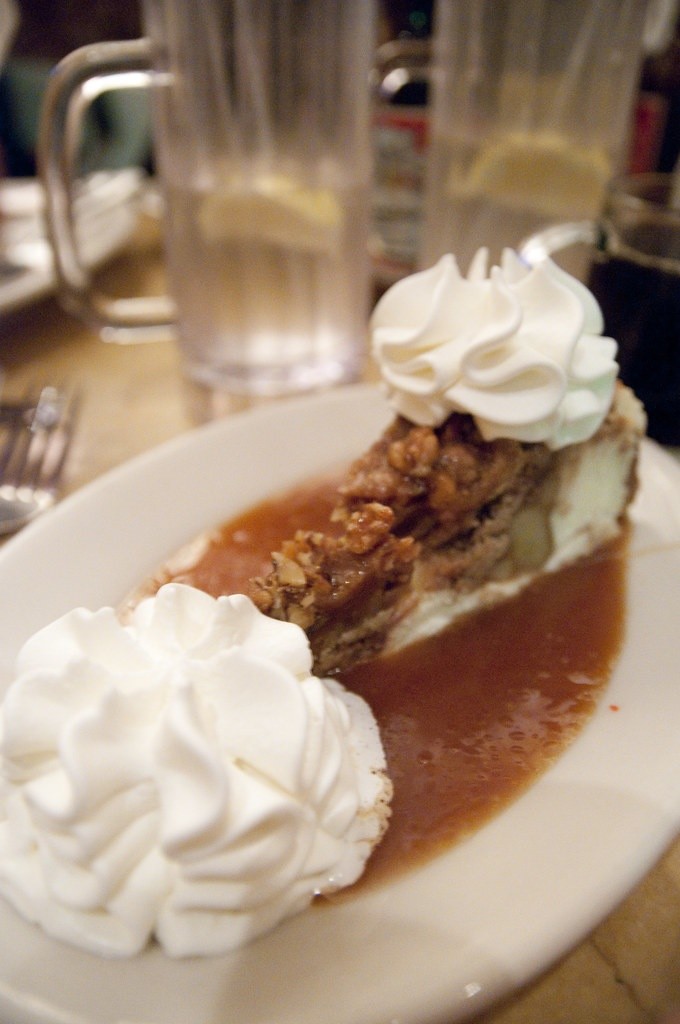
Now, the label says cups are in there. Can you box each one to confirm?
[367,1,647,295]
[591,174,680,447]
[38,0,376,395]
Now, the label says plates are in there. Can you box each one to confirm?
[0,170,134,318]
[0,388,680,1024]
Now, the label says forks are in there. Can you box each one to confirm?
[0,383,84,542]
[0,169,146,252]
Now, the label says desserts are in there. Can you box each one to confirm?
[0,246,646,955]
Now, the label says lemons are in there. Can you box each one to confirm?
[203,176,345,252]
[457,134,609,214]
